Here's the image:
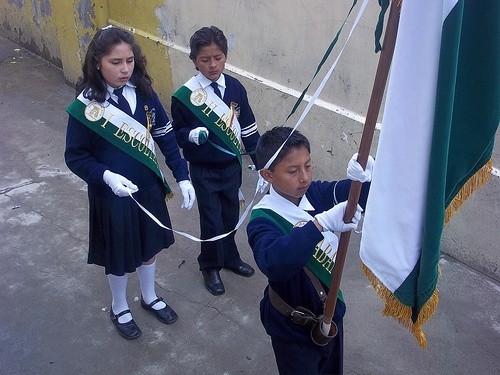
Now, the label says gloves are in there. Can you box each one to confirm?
[178,179,196,210]
[103,170,139,197]
[346,153,375,183]
[188,127,209,145]
[315,199,364,232]
[258,170,270,193]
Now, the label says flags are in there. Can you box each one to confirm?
[359,0,500,348]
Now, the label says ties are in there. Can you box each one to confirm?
[113,87,133,117]
[211,82,222,99]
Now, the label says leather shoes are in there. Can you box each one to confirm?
[201,268,225,296]
[110,306,142,339]
[140,293,178,324]
[229,260,255,276]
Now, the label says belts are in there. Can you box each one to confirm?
[268,284,322,325]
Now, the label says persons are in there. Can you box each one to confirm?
[171,26,269,295]
[64,25,195,339]
[246,126,375,375]
